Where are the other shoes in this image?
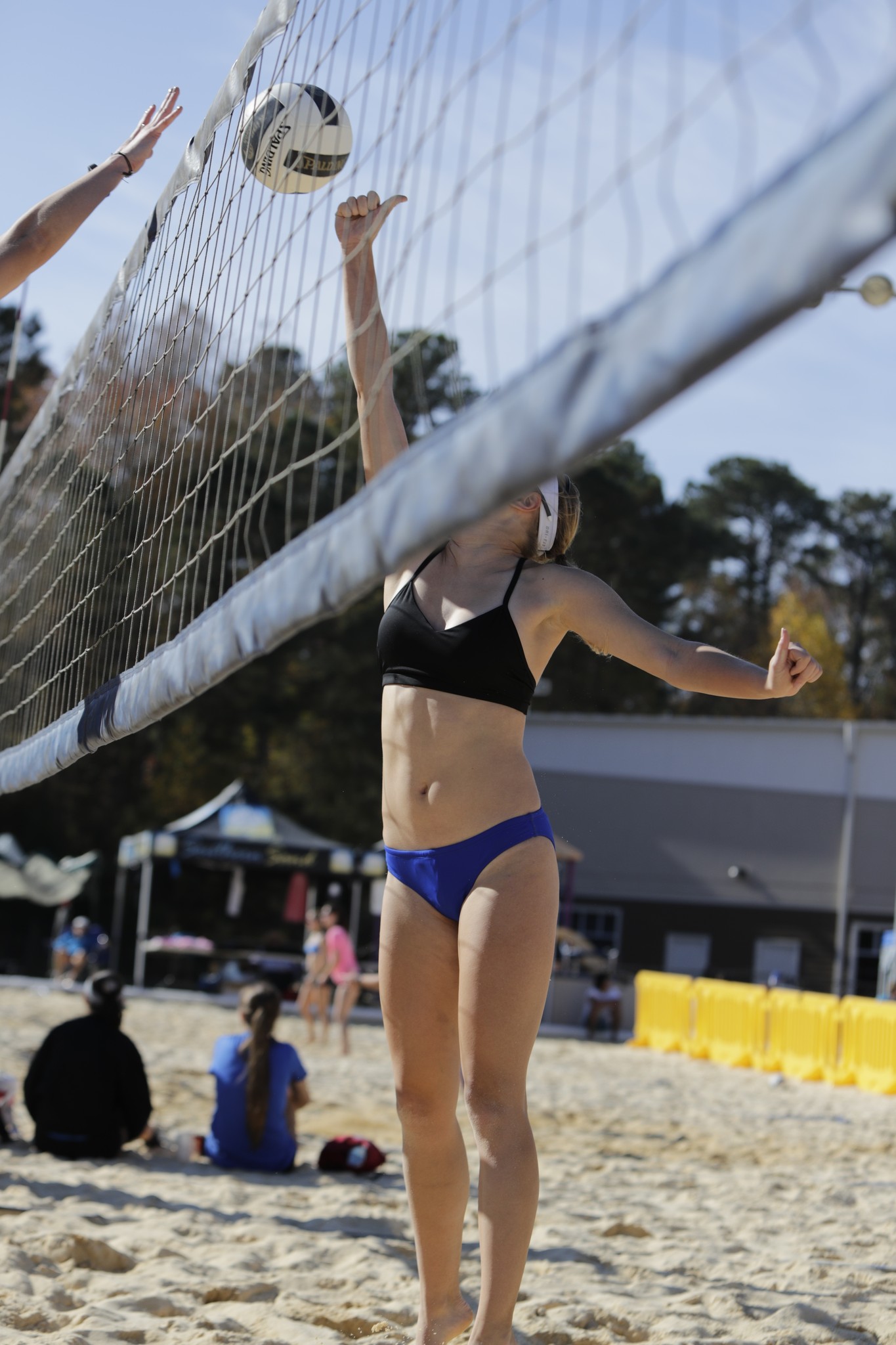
[195,1136,206,1155]
[145,1126,161,1148]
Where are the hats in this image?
[84,969,124,1010]
[535,475,559,553]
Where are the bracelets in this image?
[87,164,112,197]
[114,152,134,183]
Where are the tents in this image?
[0,776,382,994]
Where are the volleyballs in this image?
[240,80,354,196]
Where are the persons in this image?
[0,901,621,1174]
[335,191,823,1345]
[0,86,183,299]
